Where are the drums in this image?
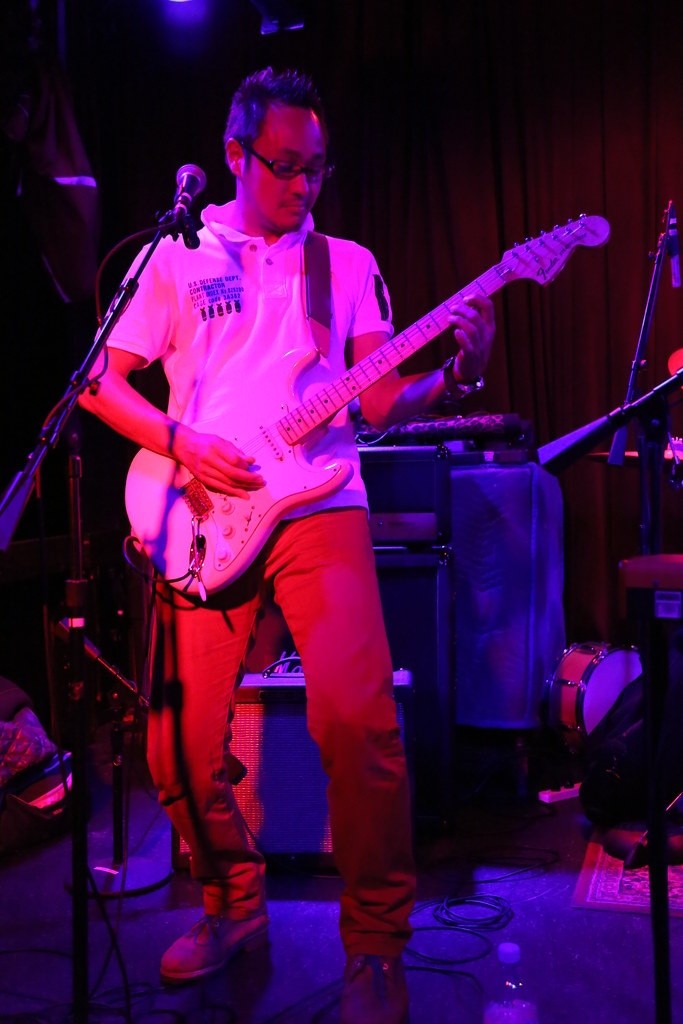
[547,639,649,755]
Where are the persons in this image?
[77,66,495,1024]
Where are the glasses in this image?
[240,141,331,180]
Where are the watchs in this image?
[442,355,486,404]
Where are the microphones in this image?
[173,163,207,220]
[666,205,681,288]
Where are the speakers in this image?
[172,462,566,875]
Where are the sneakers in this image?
[341,954,408,1024]
[160,905,269,985]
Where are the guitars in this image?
[123,209,613,601]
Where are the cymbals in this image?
[586,448,683,470]
